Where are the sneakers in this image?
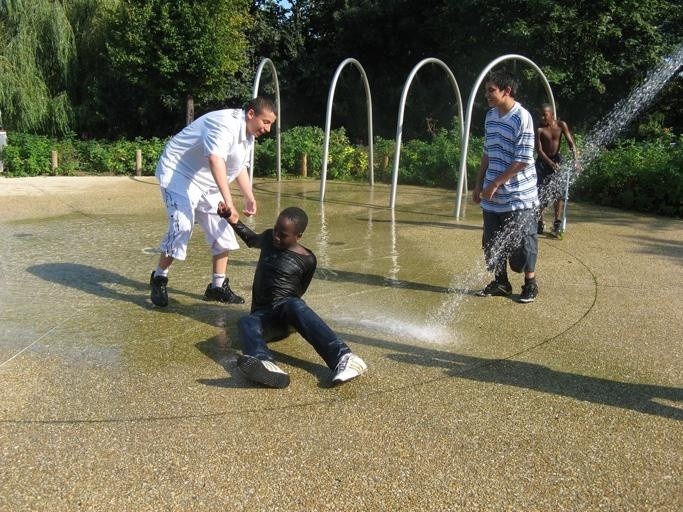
[202,277,245,304]
[537,221,544,234]
[331,353,368,387]
[517,279,539,304]
[553,220,561,233]
[236,353,291,390]
[474,280,512,297]
[149,269,169,308]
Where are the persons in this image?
[149,97,278,305]
[535,103,581,240]
[217,199,370,389]
[472,69,541,303]
